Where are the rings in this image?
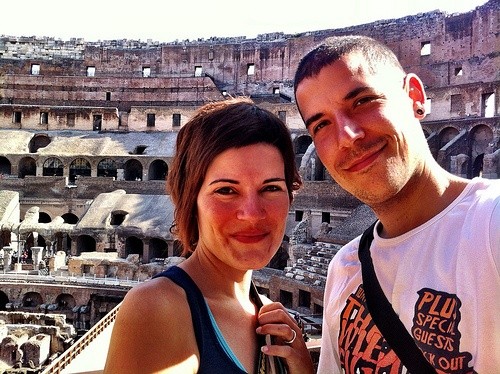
[284,327,297,345]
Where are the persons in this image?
[98,99,317,373]
[293,32,500,374]
